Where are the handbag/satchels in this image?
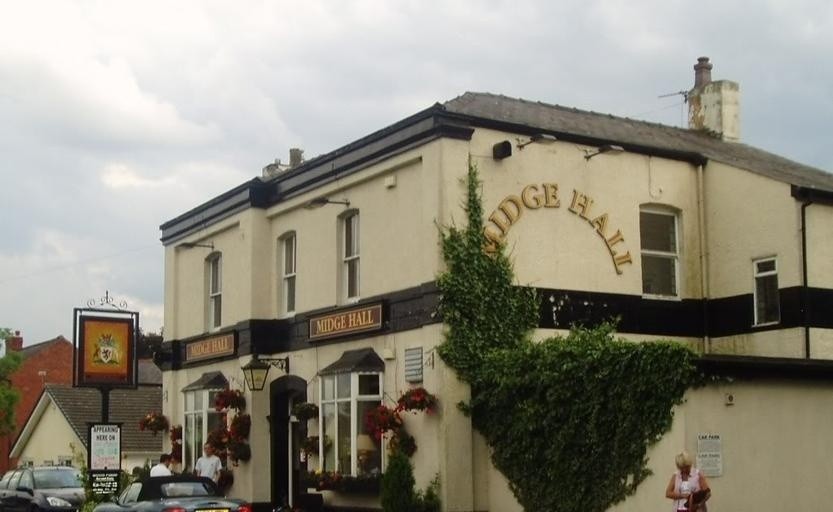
[683,488,709,512]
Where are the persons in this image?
[149,453,182,478]
[666,451,711,512]
[195,442,223,485]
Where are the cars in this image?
[0,467,88,512]
[93,474,252,512]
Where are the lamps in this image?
[241,354,290,391]
[302,198,351,208]
[174,242,214,251]
[584,144,624,162]
[517,134,557,150]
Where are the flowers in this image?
[139,390,437,456]
